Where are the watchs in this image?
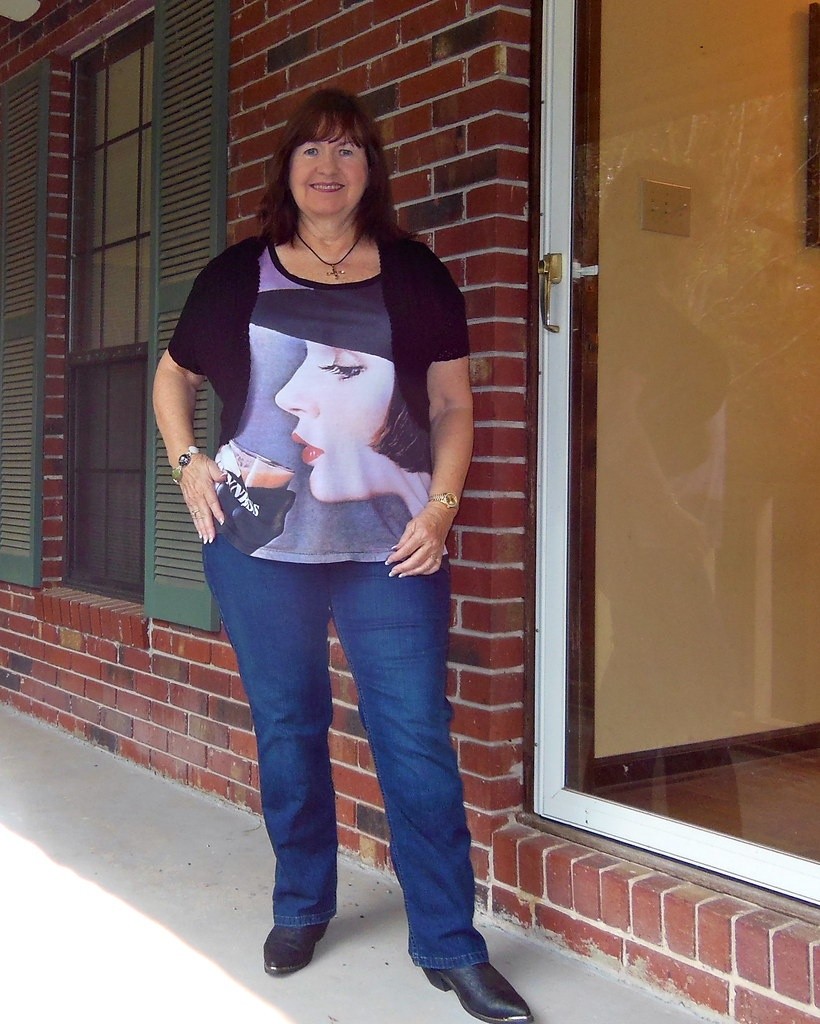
[428,492,459,511]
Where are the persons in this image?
[152,87,532,1022]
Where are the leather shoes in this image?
[421,961,535,1023]
[263,919,331,975]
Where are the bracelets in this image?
[171,444,201,484]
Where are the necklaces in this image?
[296,229,365,281]
